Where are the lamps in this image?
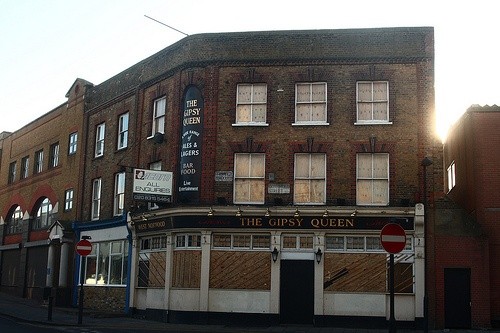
[351,209,359,217]
[265,207,272,216]
[323,209,329,217]
[294,207,300,217]
[142,212,151,221]
[236,207,243,216]
[314,247,323,264]
[271,247,278,263]
[208,207,215,216]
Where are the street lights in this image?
[418,154,432,333]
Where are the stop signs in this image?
[75,240,92,256]
[381,223,406,253]
